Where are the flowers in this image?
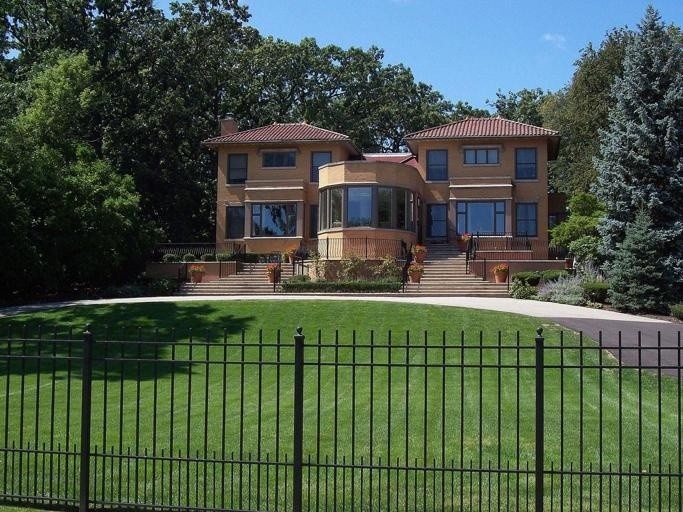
[189,265,205,272]
[411,245,427,253]
[494,263,509,271]
[265,262,282,271]
[409,261,425,271]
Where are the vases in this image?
[409,271,422,283]
[266,271,281,282]
[414,253,426,262]
[495,271,507,282]
[192,272,203,282]
